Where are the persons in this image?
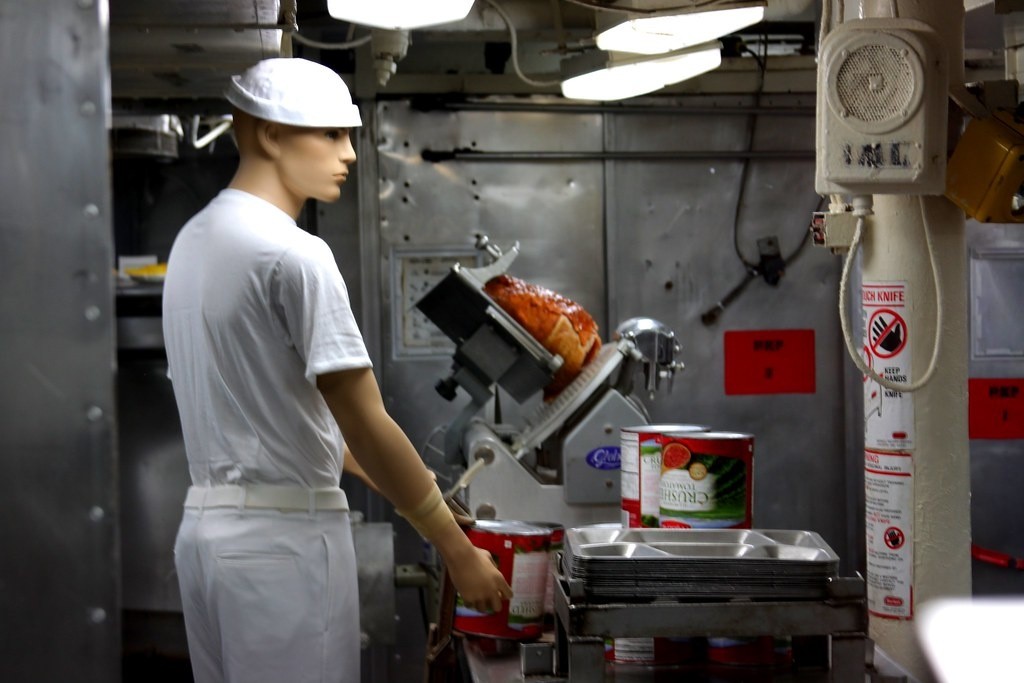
[161,55,515,683]
